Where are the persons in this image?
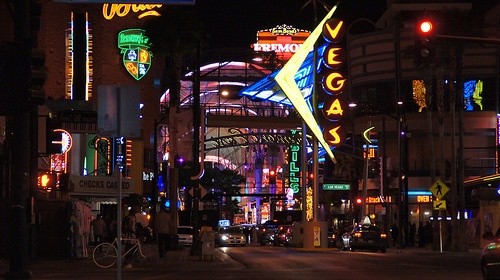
[90,205,172,260]
[383,220,433,249]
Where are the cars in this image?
[176,225,193,246]
[336,216,388,252]
[241,208,338,247]
[217,220,246,246]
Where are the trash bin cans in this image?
[201,230,215,262]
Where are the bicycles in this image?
[92,235,159,269]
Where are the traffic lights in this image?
[417,18,436,60]
